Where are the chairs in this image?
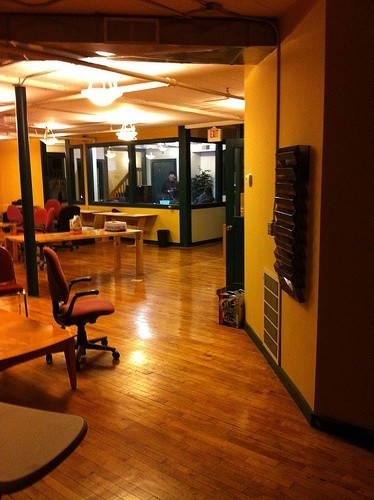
[43,246,120,372]
[3,199,81,251]
[0,246,28,318]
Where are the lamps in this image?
[81,80,123,107]
[115,123,138,141]
[39,129,58,146]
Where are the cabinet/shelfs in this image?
[126,185,153,203]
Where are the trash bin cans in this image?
[217,287,244,328]
[157,230,170,247]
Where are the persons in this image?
[193,187,214,203]
[161,172,178,200]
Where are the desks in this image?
[0,308,79,390]
[0,403,88,495]
[79,209,157,247]
[5,228,144,277]
[0,222,18,262]
